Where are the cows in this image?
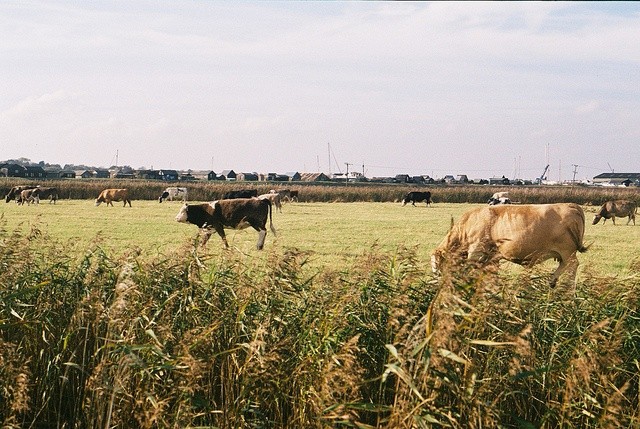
[257,193,282,213]
[94,188,132,207]
[290,190,298,203]
[432,202,594,285]
[175,199,277,250]
[36,187,58,204]
[16,188,40,206]
[268,189,290,205]
[159,187,188,203]
[591,199,638,226]
[401,191,433,208]
[6,186,39,203]
[222,188,258,198]
